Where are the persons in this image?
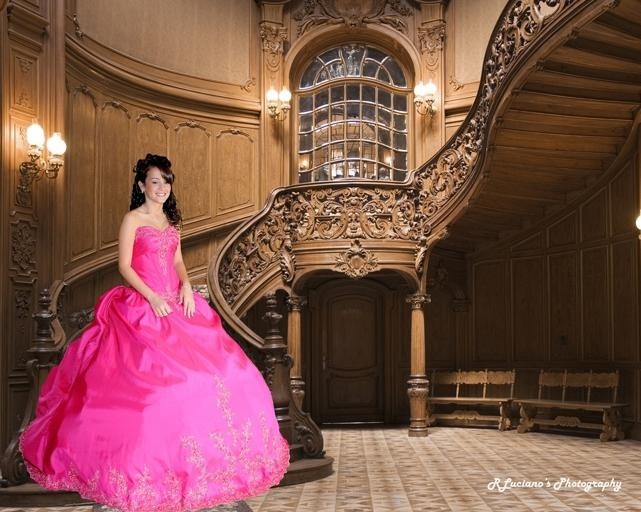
[18,154,291,511]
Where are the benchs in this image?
[428,367,517,431]
[512,370,633,441]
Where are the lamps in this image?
[266,87,291,121]
[17,123,67,193]
[413,78,437,117]
